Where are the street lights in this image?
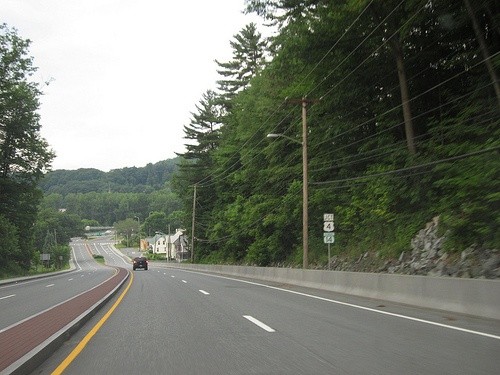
[155,231,170,261]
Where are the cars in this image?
[131,257,147,270]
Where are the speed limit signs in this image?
[323,221,334,244]
